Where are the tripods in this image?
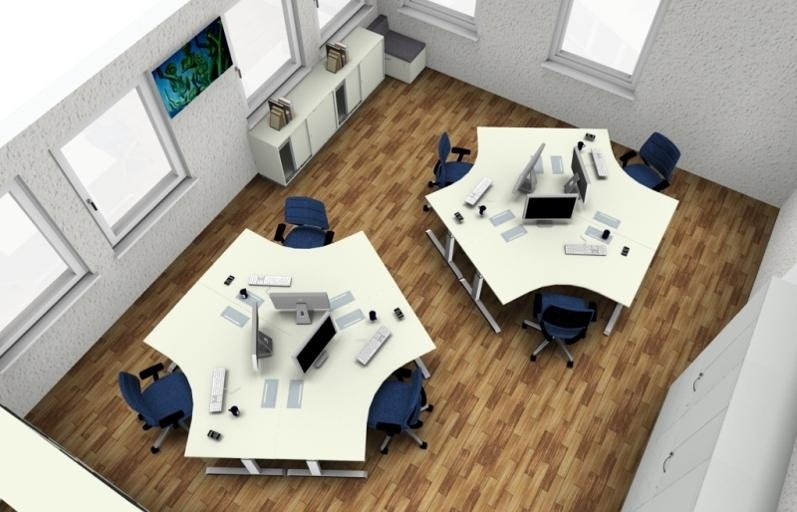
[512,142,546,195]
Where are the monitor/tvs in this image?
[251,302,273,371]
[269,292,332,325]
[521,193,579,228]
[563,146,591,204]
[291,311,337,376]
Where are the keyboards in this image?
[209,367,226,412]
[465,177,492,205]
[248,273,292,287]
[591,147,609,177]
[566,243,608,256]
[355,324,391,365]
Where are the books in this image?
[268,97,294,130]
[326,42,348,74]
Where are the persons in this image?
[156,31,219,95]
